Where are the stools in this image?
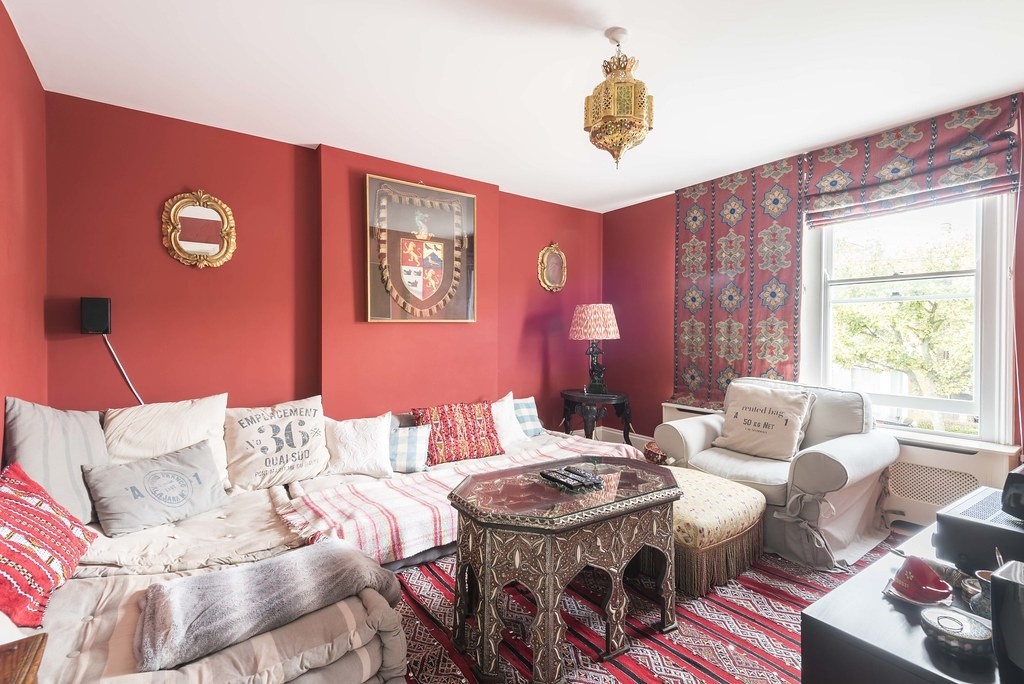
[634,465,767,597]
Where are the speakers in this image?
[79,296,112,334]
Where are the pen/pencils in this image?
[996,547,1004,567]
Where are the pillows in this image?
[712,381,816,462]
[0,461,100,630]
[5,396,111,525]
[514,396,546,436]
[388,423,432,473]
[81,439,230,537]
[224,394,331,496]
[322,410,394,478]
[491,391,531,448]
[411,399,505,467]
[99,392,232,489]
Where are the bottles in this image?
[584,385,587,394]
[969,570,993,620]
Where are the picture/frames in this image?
[364,173,477,324]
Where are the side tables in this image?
[561,388,633,447]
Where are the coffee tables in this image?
[446,456,685,684]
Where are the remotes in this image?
[541,466,605,490]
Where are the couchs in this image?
[654,376,901,574]
[0,413,652,684]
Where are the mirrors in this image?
[161,189,237,269]
[537,242,567,292]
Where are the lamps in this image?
[585,27,654,171]
[569,303,620,395]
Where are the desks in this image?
[800,519,1024,684]
